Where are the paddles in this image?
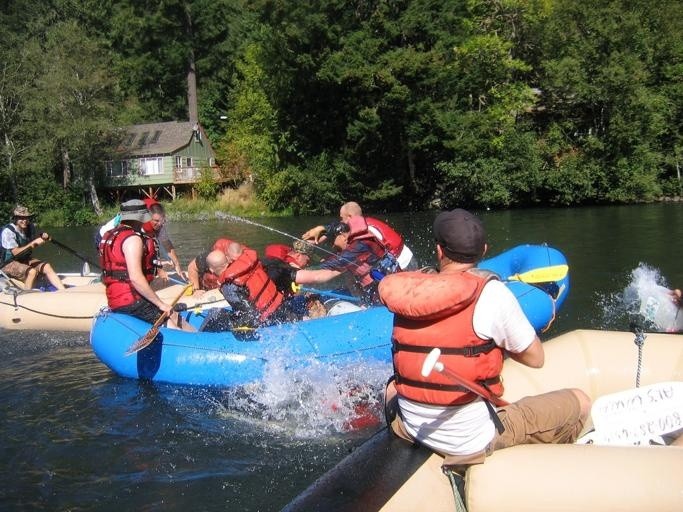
[232,326,256,333]
[125,283,195,356]
[502,264,569,283]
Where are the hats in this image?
[293,240,316,255]
[321,223,349,237]
[120,199,153,223]
[13,206,35,217]
[432,209,485,263]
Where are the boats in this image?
[270,324,683,512]
[0,266,223,335]
[86,240,572,394]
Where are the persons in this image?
[94,199,419,331]
[384,208,591,472]
[0,207,65,291]
